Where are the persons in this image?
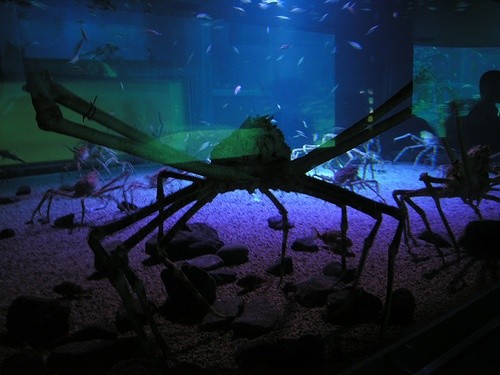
[462,70,500,161]
